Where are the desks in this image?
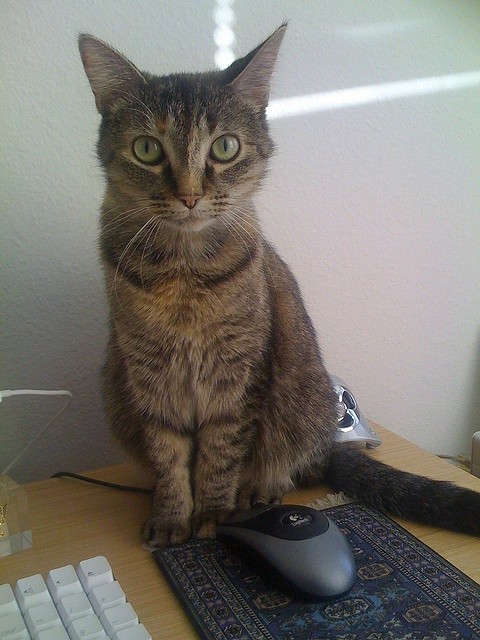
[0,418,479,639]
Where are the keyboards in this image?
[0,555,152,639]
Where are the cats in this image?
[77,21,480,548]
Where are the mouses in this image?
[216,503,359,602]
